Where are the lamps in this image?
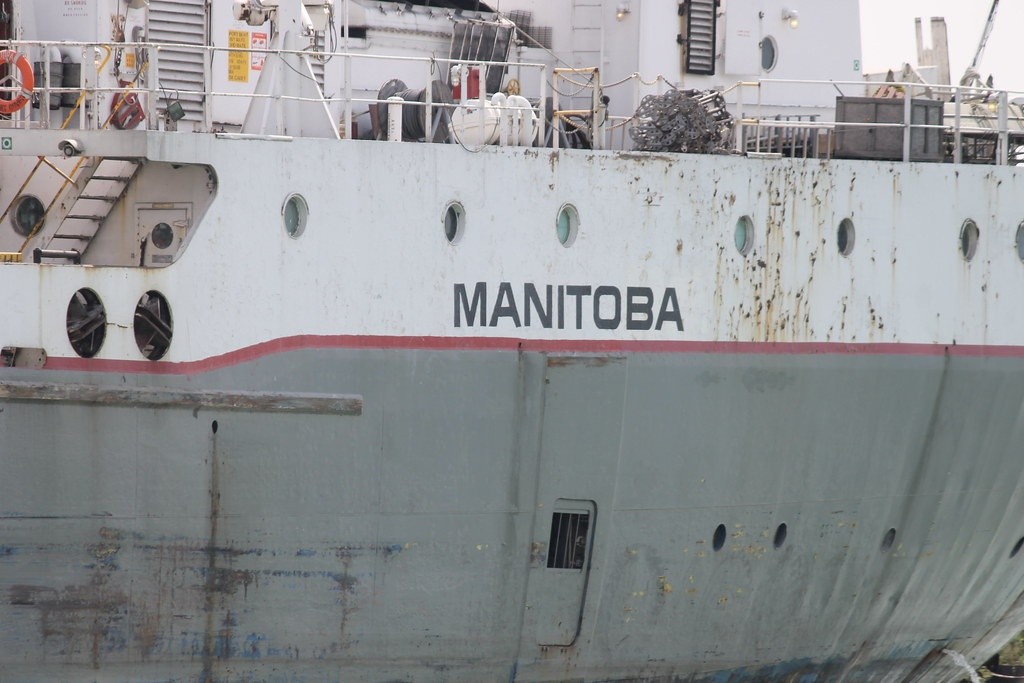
[783,8,799,28]
[165,100,184,122]
[616,2,630,17]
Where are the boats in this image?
[0,0,1024,683]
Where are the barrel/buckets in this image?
[166,91,184,121]
[62,55,81,108]
[32,61,63,110]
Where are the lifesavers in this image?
[0,49,35,114]
